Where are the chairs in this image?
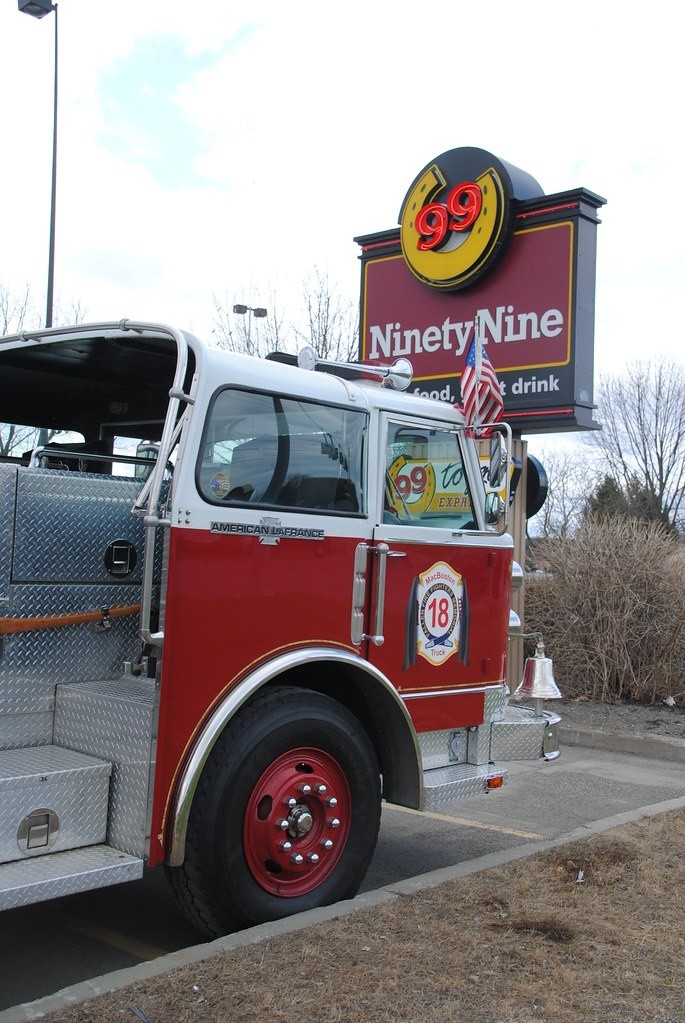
[19,441,109,474]
[233,438,345,508]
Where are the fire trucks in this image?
[0,321,563,947]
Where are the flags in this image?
[459,327,504,436]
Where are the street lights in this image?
[16,0,61,448]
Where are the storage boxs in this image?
[0,745,114,865]
